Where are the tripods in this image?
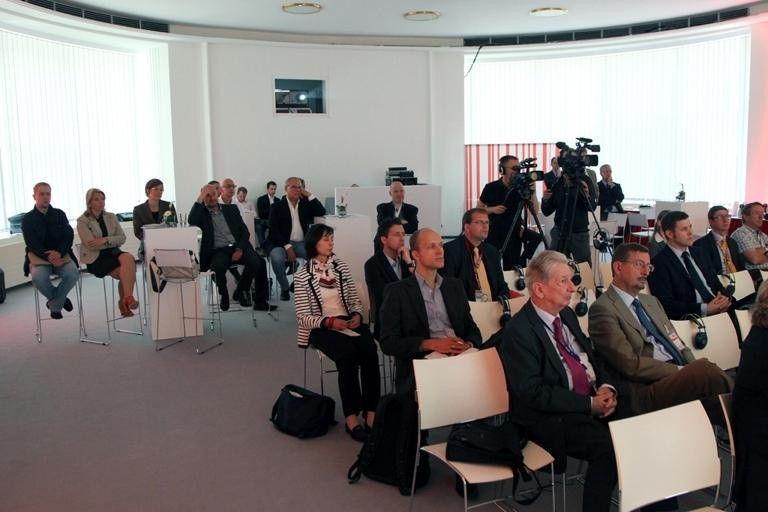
[498,201,550,267]
[556,183,614,257]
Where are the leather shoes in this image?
[279,281,294,299]
[232,289,252,307]
[455,473,478,501]
[46,301,62,319]
[118,296,139,315]
[345,421,373,442]
[220,293,229,311]
[63,297,73,311]
[253,301,277,311]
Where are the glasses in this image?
[712,215,732,219]
[622,260,655,272]
[152,187,164,192]
[474,219,490,225]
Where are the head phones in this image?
[680,313,707,349]
[576,286,588,316]
[496,295,511,328]
[718,270,735,295]
[513,265,525,291]
[567,261,581,285]
[497,156,505,175]
[592,228,610,252]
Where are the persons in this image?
[436,208,511,304]
[494,250,678,512]
[77,189,139,318]
[588,243,737,430]
[379,229,482,500]
[327,316,336,330]
[365,218,416,322]
[375,182,420,234]
[732,278,768,512]
[133,179,178,263]
[187,178,326,311]
[541,156,624,268]
[646,202,768,320]
[21,183,80,319]
[476,156,538,270]
[293,224,381,443]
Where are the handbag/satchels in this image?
[155,250,199,278]
[273,383,335,438]
[445,422,529,465]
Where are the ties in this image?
[682,251,715,302]
[632,299,684,365]
[720,239,737,272]
[474,247,493,302]
[553,318,591,395]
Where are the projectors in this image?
[277,90,308,105]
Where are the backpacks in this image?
[357,393,430,487]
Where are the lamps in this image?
[282,2,321,14]
[530,8,568,17]
[405,10,440,21]
